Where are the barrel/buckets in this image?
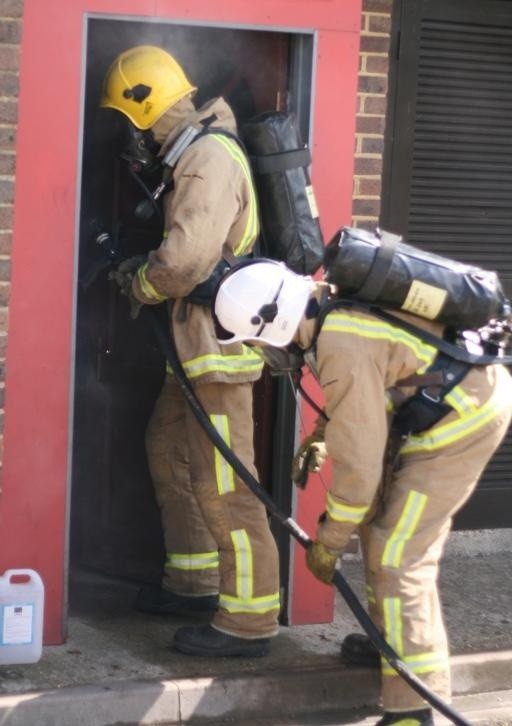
[0,569,45,666]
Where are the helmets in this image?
[99,44,197,130]
[212,256,310,348]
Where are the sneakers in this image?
[173,623,271,657]
[339,630,384,667]
[137,581,219,617]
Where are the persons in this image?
[209,256,512,726]
[95,45,281,661]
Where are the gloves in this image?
[290,424,327,489]
[304,539,342,584]
[107,255,151,321]
